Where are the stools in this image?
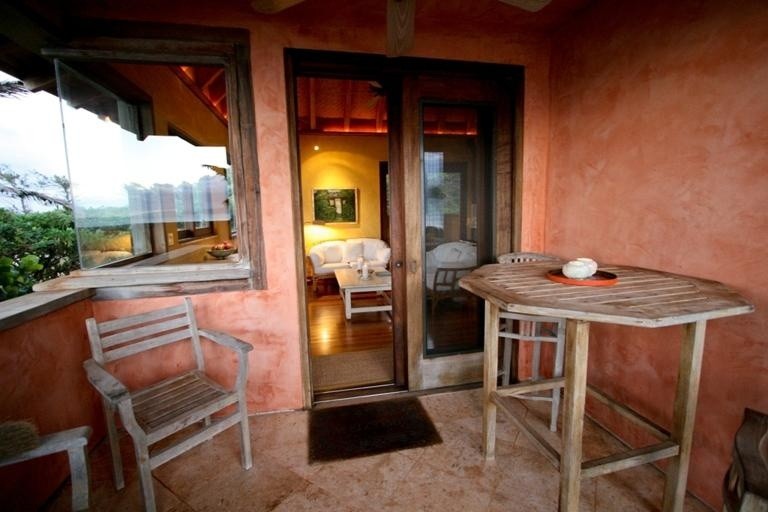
[0,425,93,512]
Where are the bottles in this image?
[356,256,369,280]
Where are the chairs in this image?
[425,265,477,322]
[496,252,566,433]
[81,296,253,512]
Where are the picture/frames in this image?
[312,188,359,224]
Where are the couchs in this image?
[305,238,392,292]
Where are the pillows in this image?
[323,239,383,262]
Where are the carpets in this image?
[307,394,443,466]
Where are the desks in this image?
[458,257,755,512]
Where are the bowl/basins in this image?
[206,247,237,260]
[574,257,598,278]
[559,260,588,280]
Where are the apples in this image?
[211,240,233,250]
